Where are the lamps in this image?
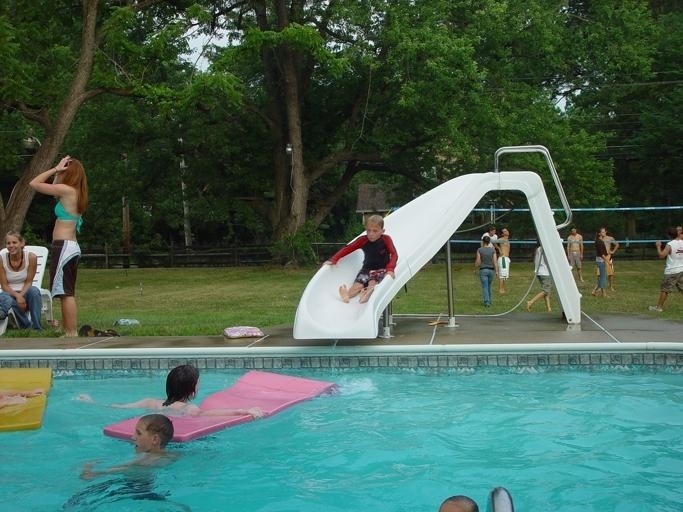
[285,143,293,153]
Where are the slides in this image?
[292,172,494,339]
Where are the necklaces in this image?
[8,254,24,271]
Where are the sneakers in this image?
[647,305,662,313]
[526,300,532,312]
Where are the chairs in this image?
[0,245,53,336]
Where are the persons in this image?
[476,224,619,312]
[437,496,480,511]
[324,215,398,302]
[30,156,87,337]
[648,226,683,312]
[81,414,193,480]
[0,230,41,332]
[77,364,265,420]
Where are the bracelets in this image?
[54,167,59,173]
